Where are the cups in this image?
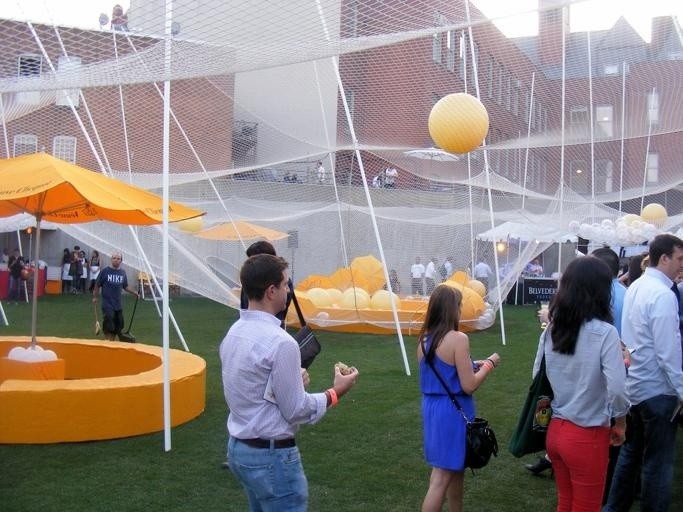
[540,300,550,308]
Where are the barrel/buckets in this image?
[0,271,17,300]
[24,265,46,298]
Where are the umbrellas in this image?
[0,147,207,349]
[195,220,291,288]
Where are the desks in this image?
[522,275,559,305]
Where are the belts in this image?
[240,436,297,449]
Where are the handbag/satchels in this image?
[465,416,498,470]
[293,324,321,369]
[509,370,553,459]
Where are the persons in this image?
[222,241,294,472]
[385,163,398,189]
[377,167,386,188]
[316,161,325,186]
[91,252,140,340]
[373,176,379,188]
[390,256,495,300]
[1,248,9,263]
[60,245,101,295]
[7,249,25,306]
[526,234,681,512]
[218,253,359,511]
[417,284,500,510]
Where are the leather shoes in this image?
[524,456,552,474]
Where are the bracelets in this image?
[327,387,340,410]
[484,359,495,372]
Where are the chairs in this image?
[161,273,180,296]
[137,272,157,297]
